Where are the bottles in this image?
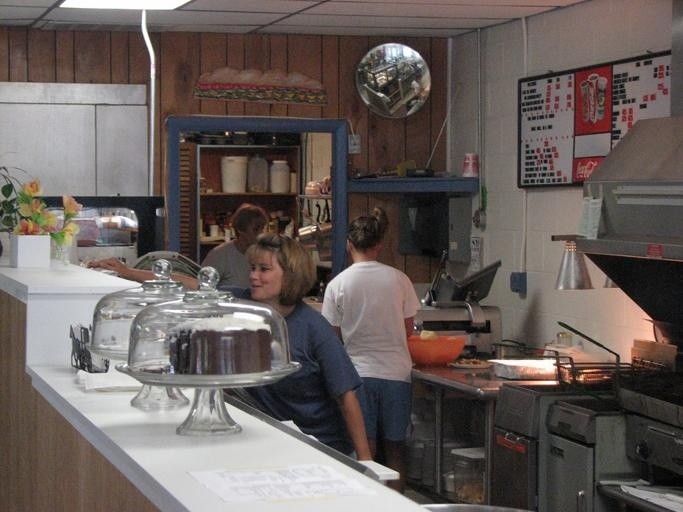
[247,155,268,192]
[270,160,290,194]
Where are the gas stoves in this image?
[618,358,683,407]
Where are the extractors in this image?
[552,117,682,323]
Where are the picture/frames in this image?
[518,49,672,190]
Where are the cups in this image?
[580,73,608,123]
[462,153,479,178]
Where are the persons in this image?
[320,206,421,493]
[88,234,371,460]
[411,73,425,96]
[199,203,268,287]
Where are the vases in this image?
[9,233,51,268]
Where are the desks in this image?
[404,352,613,506]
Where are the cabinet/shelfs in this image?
[194,143,301,267]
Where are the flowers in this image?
[12,178,83,249]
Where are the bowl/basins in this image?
[407,335,468,366]
